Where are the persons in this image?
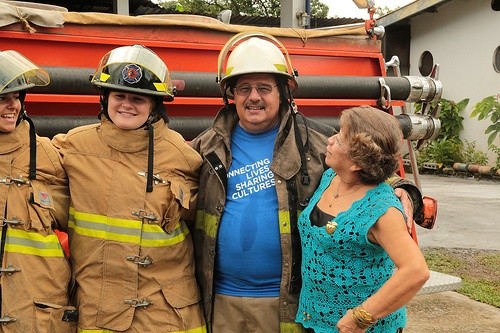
[191,32,424,332]
[0,49,72,332]
[293,105,431,333]
[51,44,207,333]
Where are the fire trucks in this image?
[0,0,444,258]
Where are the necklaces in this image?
[328,180,365,207]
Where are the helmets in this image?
[0,51,36,94]
[90,44,176,103]
[216,29,299,101]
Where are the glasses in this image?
[232,80,279,95]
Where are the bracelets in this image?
[351,304,379,330]
